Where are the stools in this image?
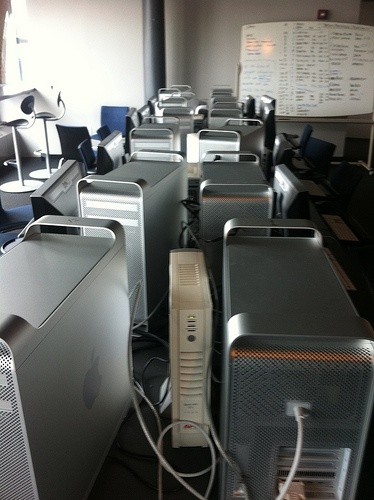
[0,95,44,194]
[28,89,67,181]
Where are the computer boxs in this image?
[3,83,373,499]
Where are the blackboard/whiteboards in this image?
[234,20,374,125]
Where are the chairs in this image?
[298,159,363,219]
[90,105,130,145]
[292,136,337,185]
[0,195,34,235]
[78,137,101,175]
[55,123,94,176]
[286,124,314,160]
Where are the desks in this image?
[0,84,36,102]
